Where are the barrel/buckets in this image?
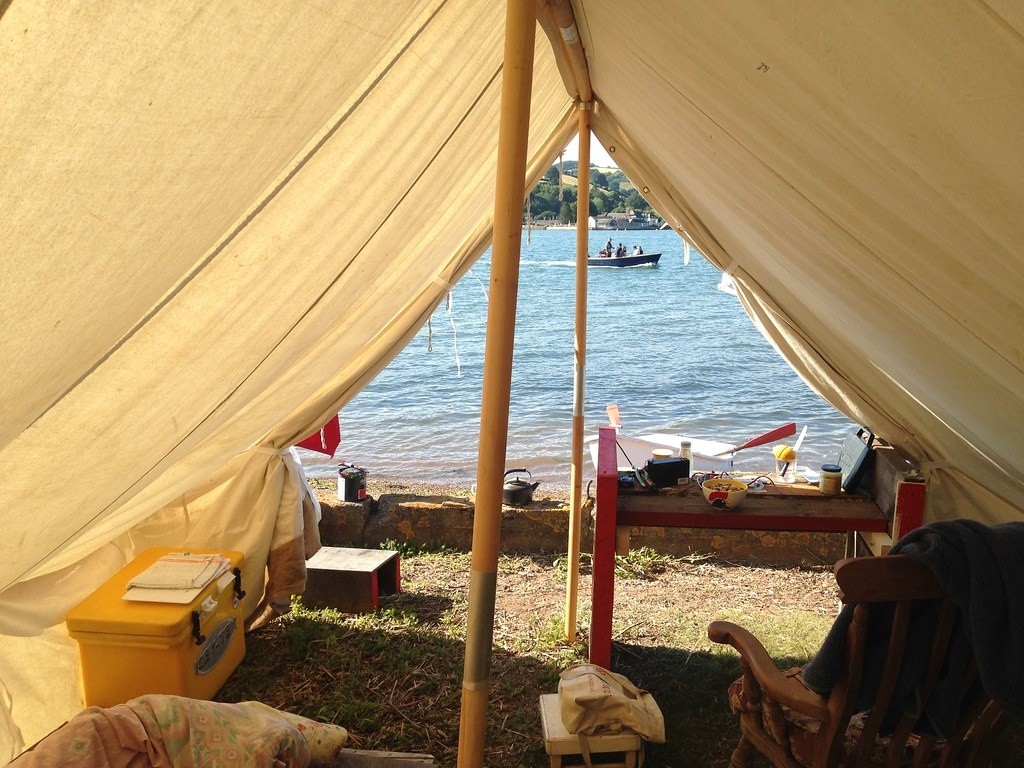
[337,463,369,503]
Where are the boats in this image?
[581,238,660,268]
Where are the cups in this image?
[606,404,620,425]
[651,448,674,460]
[775,455,799,484]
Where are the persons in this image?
[615,243,643,257]
[605,237,615,257]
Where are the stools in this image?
[303,547,402,614]
[539,695,641,768]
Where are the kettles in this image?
[502,467,540,505]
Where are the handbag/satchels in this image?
[557,663,665,768]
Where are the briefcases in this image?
[837,425,875,494]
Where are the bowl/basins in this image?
[702,478,748,510]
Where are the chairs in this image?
[707,553,1024,768]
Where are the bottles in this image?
[677,441,693,480]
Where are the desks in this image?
[590,428,923,672]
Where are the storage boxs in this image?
[67,548,250,711]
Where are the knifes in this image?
[778,425,808,477]
